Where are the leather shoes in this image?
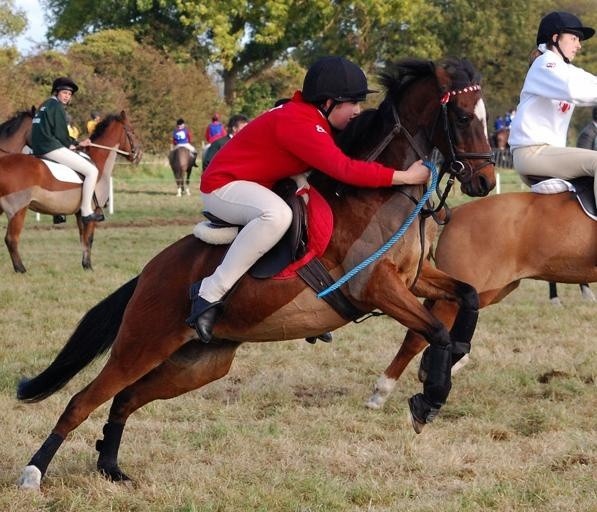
[80,212,104,223]
[190,279,219,344]
[317,332,332,343]
[53,215,66,225]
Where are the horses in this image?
[1,106,142,273]
[166,144,198,197]
[362,178,596,409]
[14,58,494,495]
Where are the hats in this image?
[51,77,79,95]
[302,56,380,103]
[536,10,595,44]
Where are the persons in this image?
[188,55,432,344]
[507,11,597,208]
[87,109,101,138]
[504,108,515,129]
[65,112,79,140]
[172,118,199,168]
[576,105,597,151]
[202,113,227,164]
[31,76,105,224]
[202,115,250,172]
[490,115,504,149]
